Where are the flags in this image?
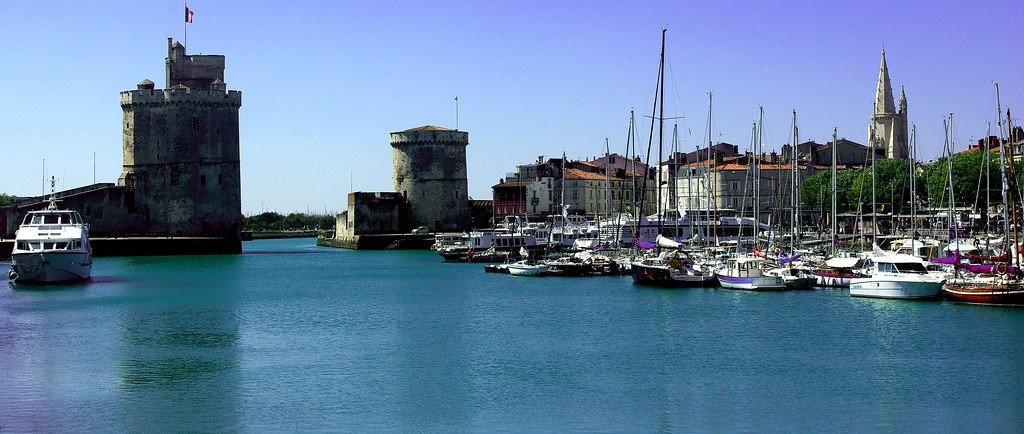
[185,7,194,24]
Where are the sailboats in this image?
[431,23,1024,308]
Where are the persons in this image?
[467,248,473,258]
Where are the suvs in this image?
[412,225,429,235]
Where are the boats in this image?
[7,158,94,283]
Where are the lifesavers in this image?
[995,262,1008,275]
